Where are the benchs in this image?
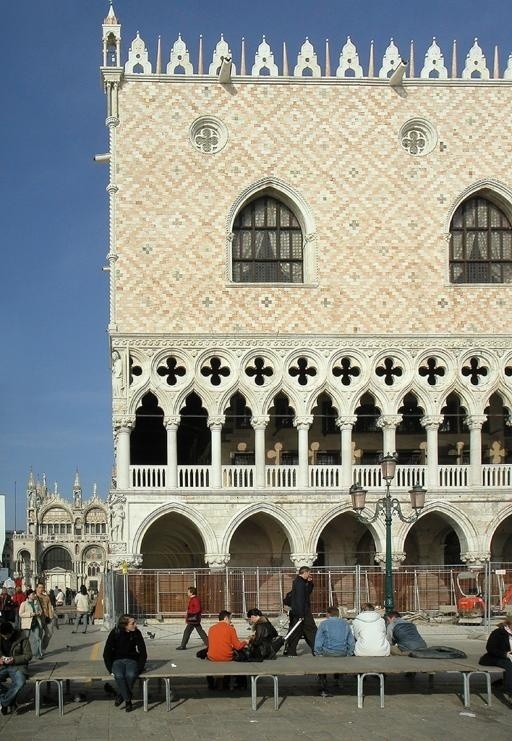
[1,651,511,714]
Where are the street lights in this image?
[349,451,429,637]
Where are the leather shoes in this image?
[176,646,185,650]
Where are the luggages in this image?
[271,618,303,651]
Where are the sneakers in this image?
[126,701,132,712]
[207,682,247,691]
[115,694,123,706]
[321,685,342,693]
[1,705,7,715]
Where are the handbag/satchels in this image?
[283,591,291,606]
[186,614,198,622]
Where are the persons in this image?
[501,585,512,622]
[314,607,355,689]
[1,622,34,716]
[282,579,298,657]
[234,609,279,692]
[485,616,512,699]
[1,577,99,662]
[351,603,390,682]
[175,587,209,652]
[385,611,428,681]
[104,613,147,713]
[207,611,251,693]
[287,567,318,657]
